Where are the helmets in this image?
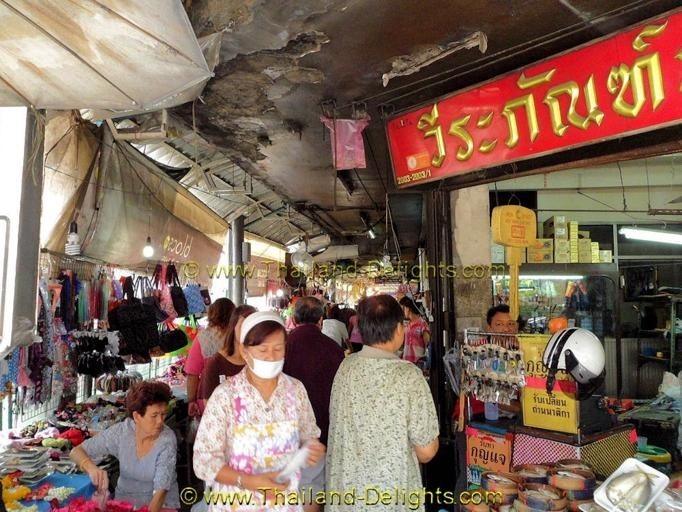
[541,326,607,402]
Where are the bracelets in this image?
[78,457,90,475]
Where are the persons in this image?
[451,303,520,512]
[69,380,181,512]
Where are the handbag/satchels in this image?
[109,263,213,364]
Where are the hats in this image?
[239,310,285,344]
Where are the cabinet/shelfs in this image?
[629,285,681,400]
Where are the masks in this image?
[244,350,285,380]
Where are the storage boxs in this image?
[22,470,95,511]
[509,418,643,481]
[465,408,518,489]
[485,210,613,268]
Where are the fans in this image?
[289,233,317,273]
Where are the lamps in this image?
[618,214,682,246]
[66,176,83,258]
[142,189,155,260]
